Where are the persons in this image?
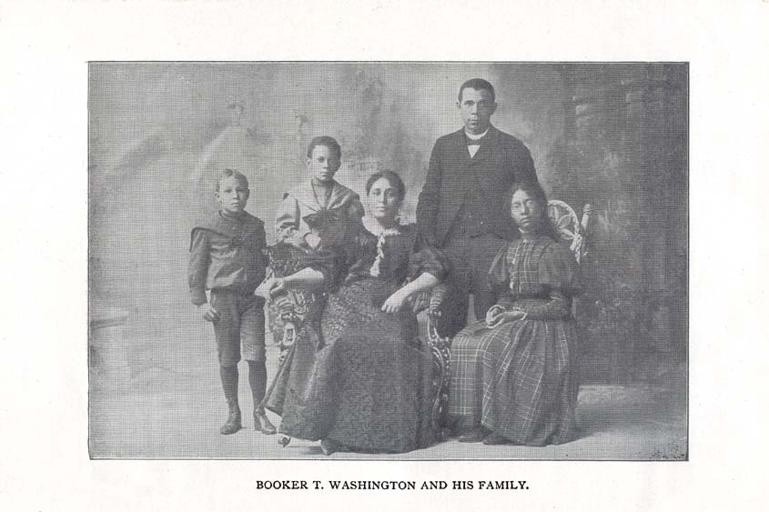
[417,79,547,337]
[261,170,452,456]
[274,136,365,254]
[446,180,586,447]
[187,169,277,435]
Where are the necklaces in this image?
[313,181,332,200]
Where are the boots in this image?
[253,399,276,434]
[220,397,240,434]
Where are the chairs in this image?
[546,197,594,321]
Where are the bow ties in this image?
[466,136,483,145]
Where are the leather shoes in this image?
[458,426,510,444]
